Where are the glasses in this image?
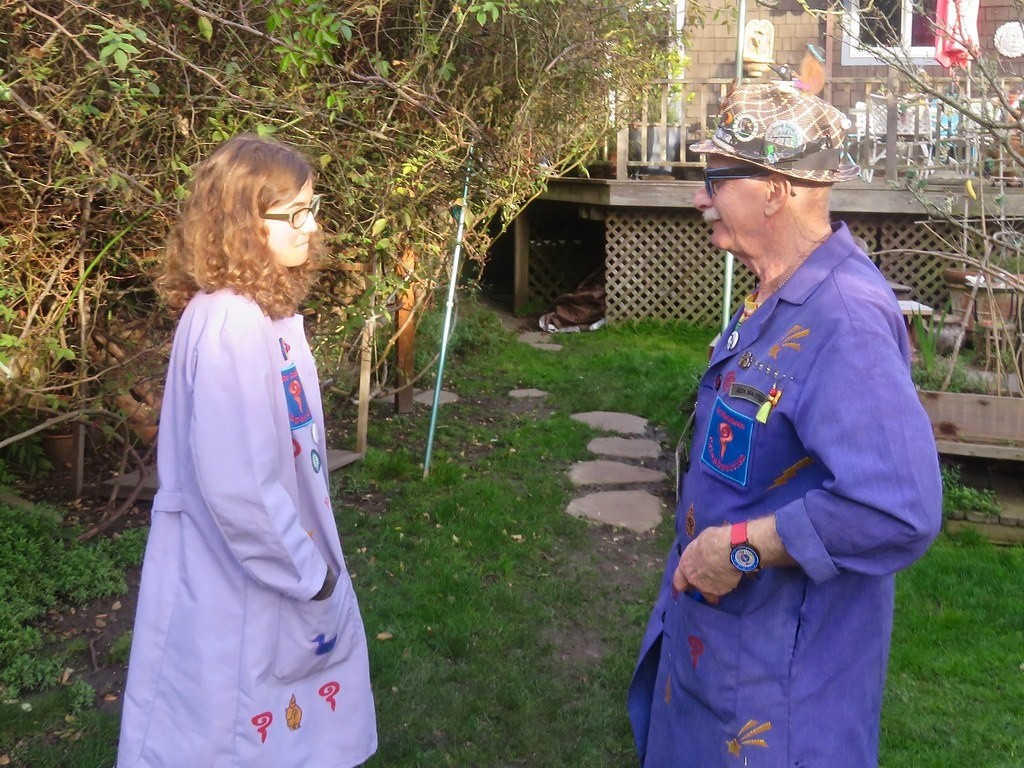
[260,195,322,228]
[702,164,797,198]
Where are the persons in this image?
[626,83,943,768]
[118,137,377,768]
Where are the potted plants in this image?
[625,95,697,175]
[950,485,1000,524]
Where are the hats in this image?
[688,78,861,183]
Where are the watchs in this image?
[729,523,764,574]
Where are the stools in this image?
[970,320,1015,372]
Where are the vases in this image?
[577,137,629,180]
[41,432,74,468]
[923,267,1024,358]
[680,141,706,181]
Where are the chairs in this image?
[855,94,1002,185]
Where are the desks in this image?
[888,282,932,363]
